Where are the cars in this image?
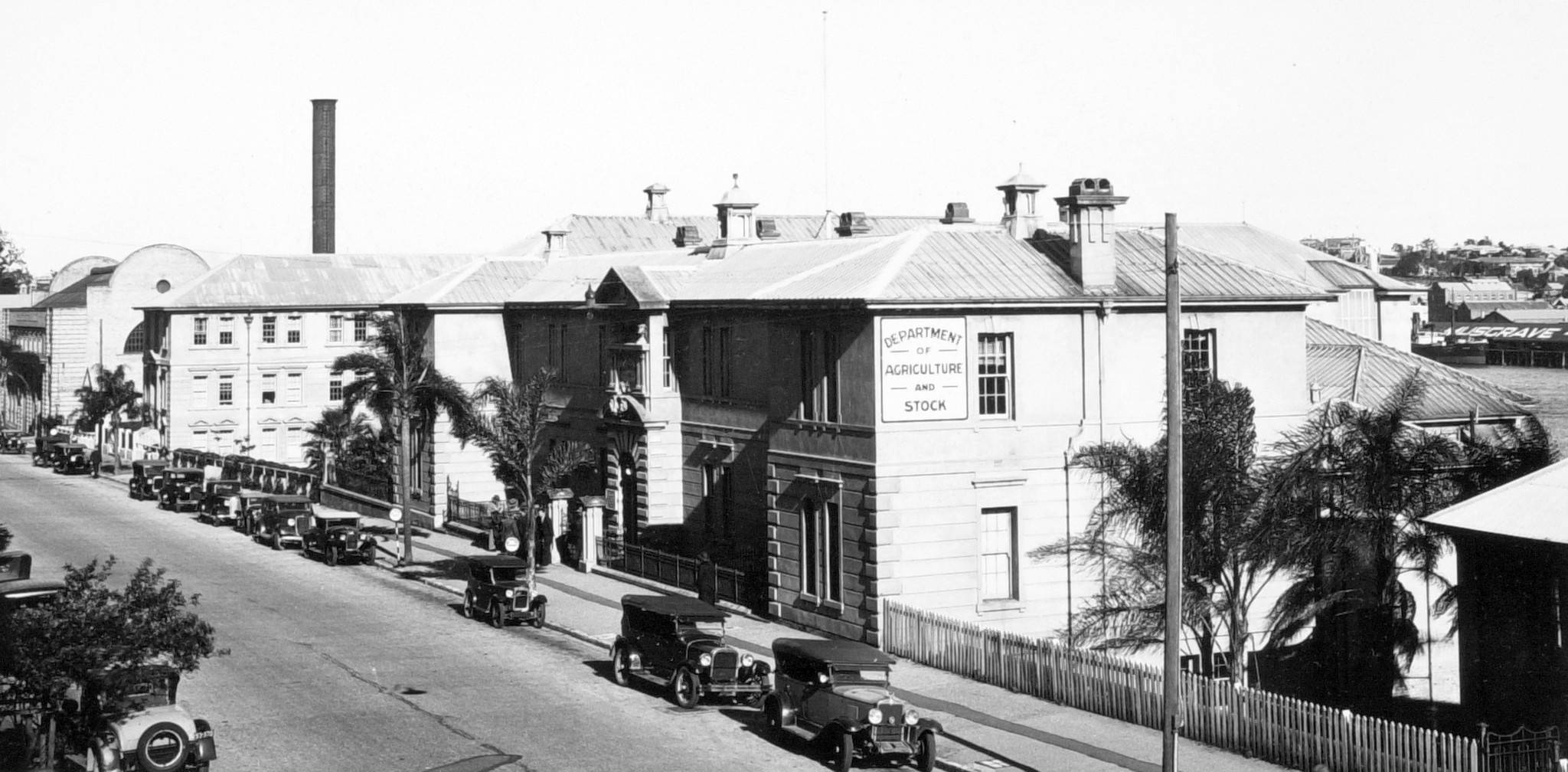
[0,578,73,617]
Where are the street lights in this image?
[243,312,254,456]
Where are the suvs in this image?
[253,495,317,551]
[59,649,219,772]
[129,458,174,500]
[158,467,209,513]
[0,429,26,454]
[462,555,548,629]
[31,436,67,468]
[301,510,378,567]
[196,479,247,527]
[51,443,94,475]
[608,594,773,709]
[764,638,944,772]
[234,492,274,536]
